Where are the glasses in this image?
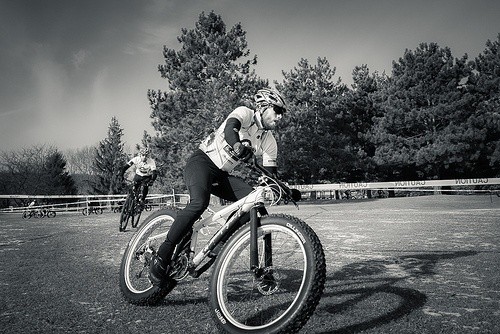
[273,105,285,116]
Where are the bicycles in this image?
[82,203,103,216]
[113,203,123,214]
[115,174,154,232]
[22,208,57,220]
[118,145,327,334]
[144,202,153,212]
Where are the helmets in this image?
[254,87,287,115]
[139,148,149,155]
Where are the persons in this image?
[120,148,157,206]
[148,88,301,290]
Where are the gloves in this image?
[234,142,253,163]
[288,188,302,203]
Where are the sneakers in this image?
[148,252,170,288]
